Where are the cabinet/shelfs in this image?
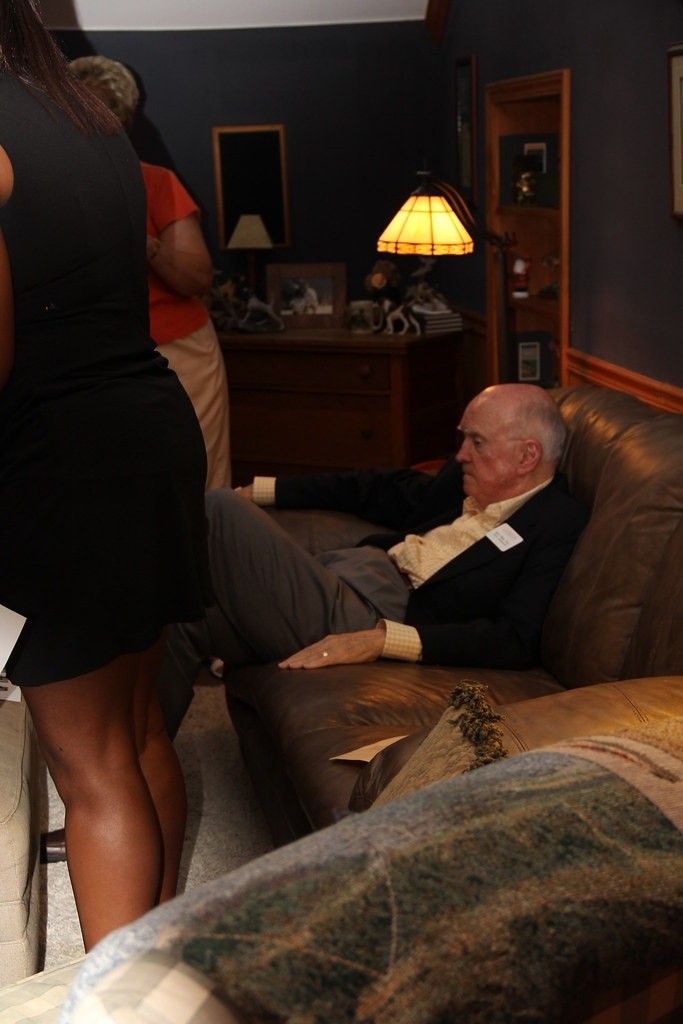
[220,330,454,475]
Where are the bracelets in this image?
[147,239,162,263]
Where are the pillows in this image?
[367,679,509,811]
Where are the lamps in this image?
[370,169,520,373]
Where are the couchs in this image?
[65,387,683,1024]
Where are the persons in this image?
[64,54,232,488]
[1,0,224,959]
[289,277,321,314]
[159,384,593,743]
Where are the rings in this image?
[323,650,330,657]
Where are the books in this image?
[408,307,464,335]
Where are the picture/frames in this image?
[215,126,291,252]
[268,262,349,330]
[667,44,683,216]
